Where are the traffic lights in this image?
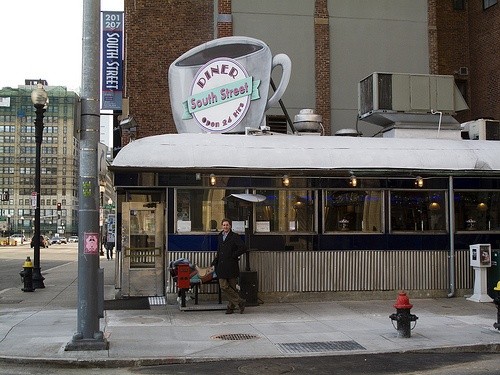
[57,203,61,211]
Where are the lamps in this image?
[415,175,424,188]
[350,175,357,186]
[283,175,290,186]
[210,174,216,186]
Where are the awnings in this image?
[108,133,500,175]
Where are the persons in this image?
[211,219,245,314]
[104,233,115,261]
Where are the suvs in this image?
[30,234,49,248]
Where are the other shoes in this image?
[238,300,247,314]
[225,305,235,314]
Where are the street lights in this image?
[30,77,48,290]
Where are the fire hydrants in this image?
[389,290,418,339]
[19,257,35,292]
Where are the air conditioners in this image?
[458,67,469,76]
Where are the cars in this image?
[6,234,27,243]
[48,235,79,245]
